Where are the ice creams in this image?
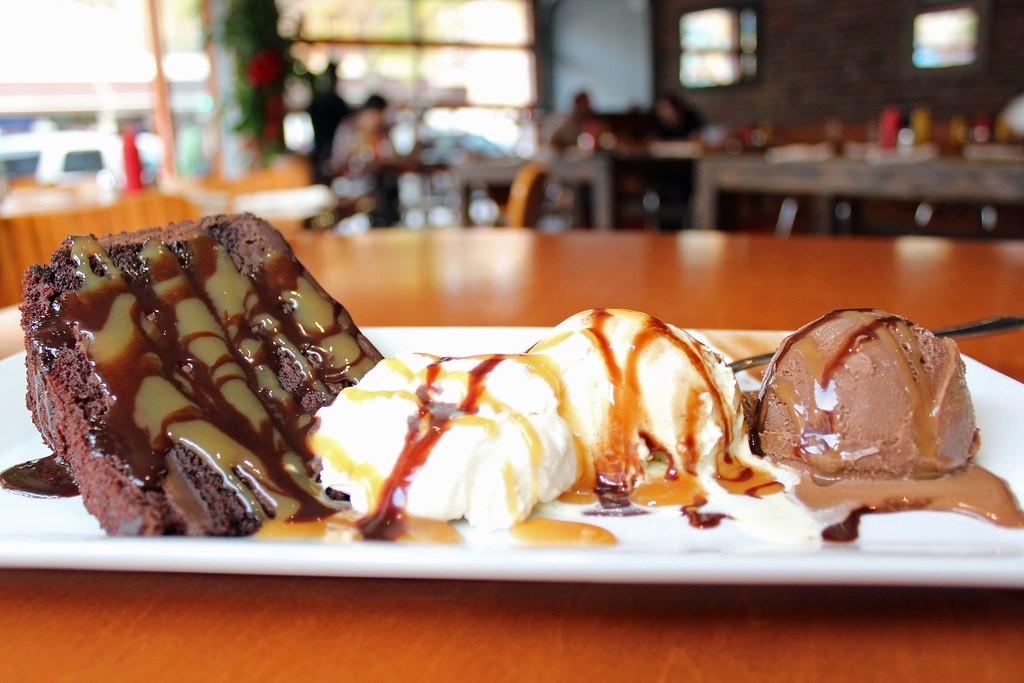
[303,309,981,529]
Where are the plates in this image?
[0,326,1024,586]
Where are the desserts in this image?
[20,208,380,540]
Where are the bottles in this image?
[875,104,1014,149]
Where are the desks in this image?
[694,150,1024,242]
[0,226,1024,683]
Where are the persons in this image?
[330,95,432,231]
[547,87,736,230]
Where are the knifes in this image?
[728,315,1022,377]
